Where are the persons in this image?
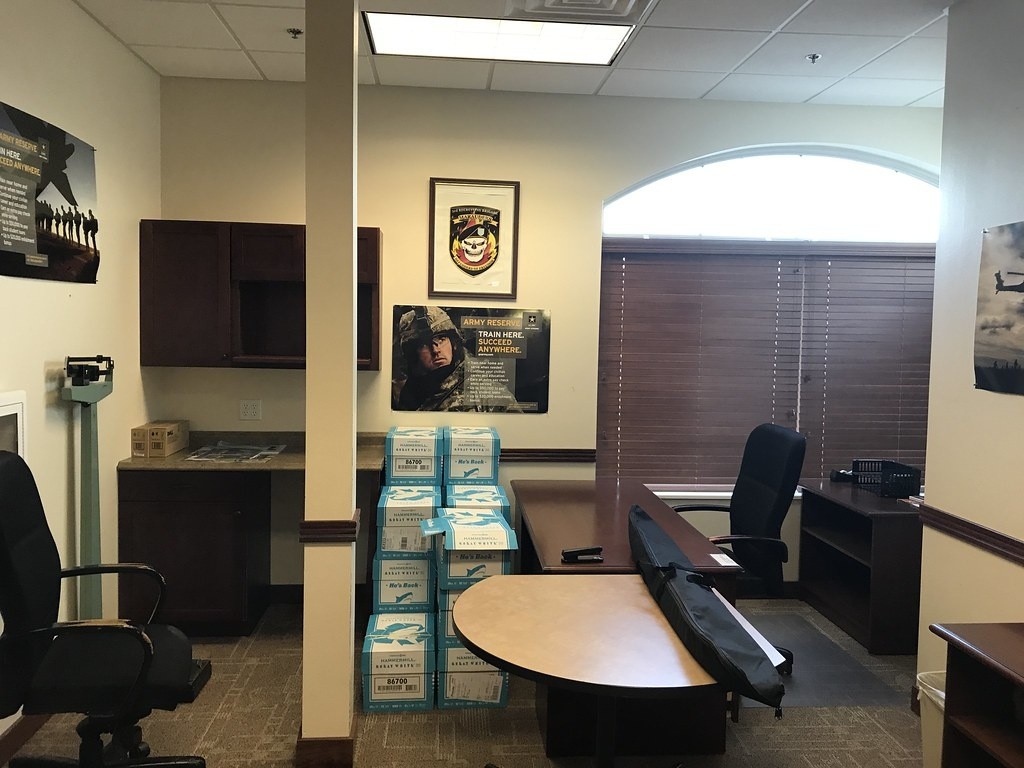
[392,306,523,413]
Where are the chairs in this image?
[0,449,212,768]
[672,422,807,676]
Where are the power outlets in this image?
[239,399,262,421]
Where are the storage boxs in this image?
[361,426,519,712]
[131,419,189,457]
[852,457,921,498]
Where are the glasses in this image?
[418,334,451,353]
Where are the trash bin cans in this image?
[915,671,947,768]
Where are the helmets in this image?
[399,306,461,358]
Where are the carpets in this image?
[736,614,906,709]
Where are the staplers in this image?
[560,545,604,563]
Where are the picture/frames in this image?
[428,177,520,299]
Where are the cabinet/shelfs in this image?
[139,218,381,371]
[798,476,923,656]
[929,622,1024,768]
[118,431,386,637]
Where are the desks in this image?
[451,575,785,768]
[508,476,747,756]
